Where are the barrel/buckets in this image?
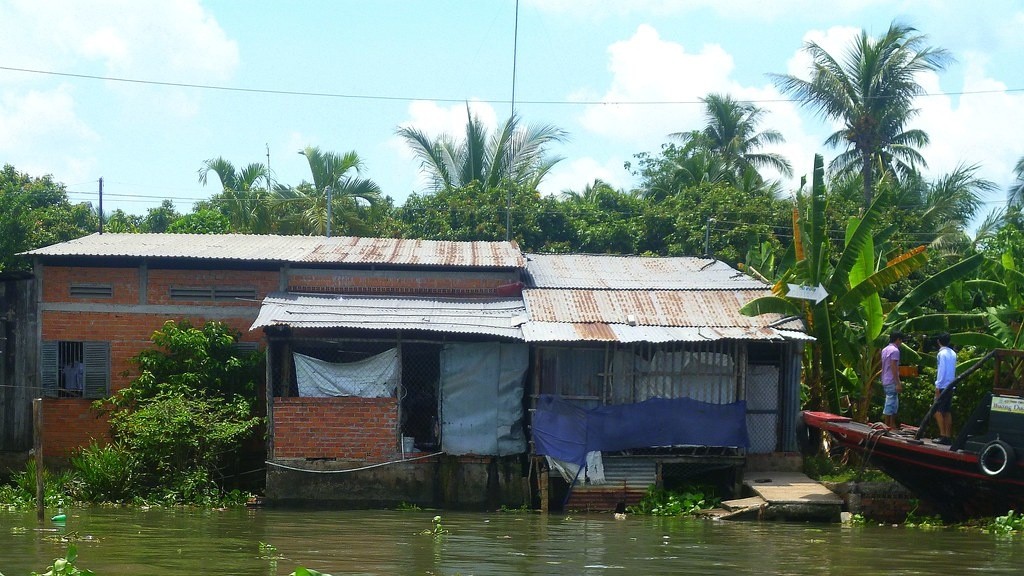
[403,436,414,453]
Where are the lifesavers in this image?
[978,439,1016,480]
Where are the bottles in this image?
[50,514,67,521]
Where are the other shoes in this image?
[943,438,952,444]
[932,436,947,444]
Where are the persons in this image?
[932,332,957,444]
[882,330,903,431]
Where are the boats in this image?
[802,347,1022,528]
